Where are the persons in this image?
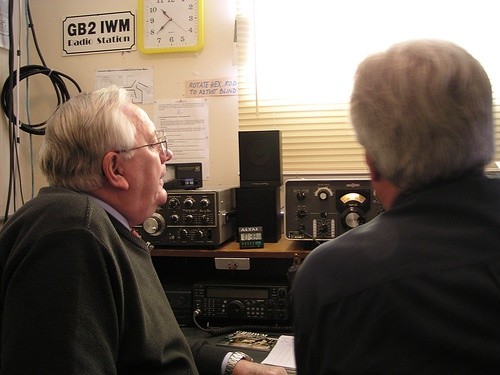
[288,37,500,375]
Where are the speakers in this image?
[238,130,283,187]
[235,187,281,243]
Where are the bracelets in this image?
[0,83,288,375]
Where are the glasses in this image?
[113,130,168,153]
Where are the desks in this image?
[151,232,312,375]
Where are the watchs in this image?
[223,351,255,375]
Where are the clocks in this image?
[137,0,205,55]
